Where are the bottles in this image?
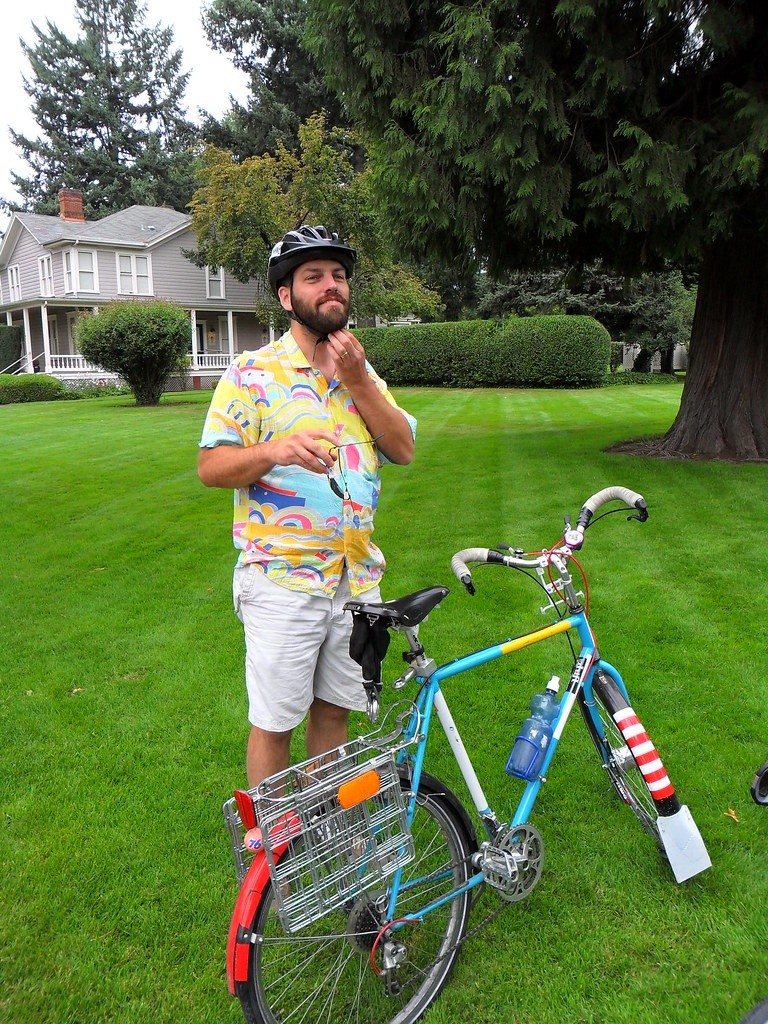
[506,675,560,780]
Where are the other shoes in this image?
[268,878,291,911]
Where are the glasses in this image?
[325,432,385,501]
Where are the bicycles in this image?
[224,483,712,1023]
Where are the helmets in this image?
[267,225,357,301]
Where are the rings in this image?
[341,351,348,358]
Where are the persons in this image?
[197,226,418,855]
